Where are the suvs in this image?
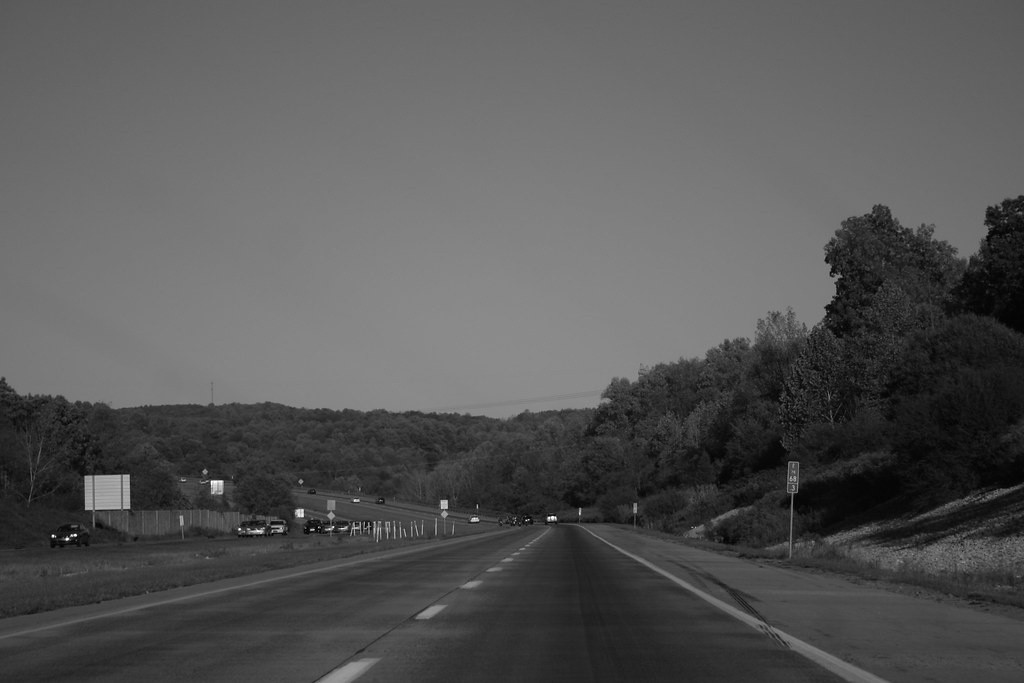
[375,497,385,504]
[352,497,360,503]
[237,518,289,538]
[468,514,480,524]
[498,513,558,526]
[307,488,316,495]
[177,477,187,483]
[198,478,209,485]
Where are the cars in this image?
[49,522,91,549]
[303,519,375,535]
